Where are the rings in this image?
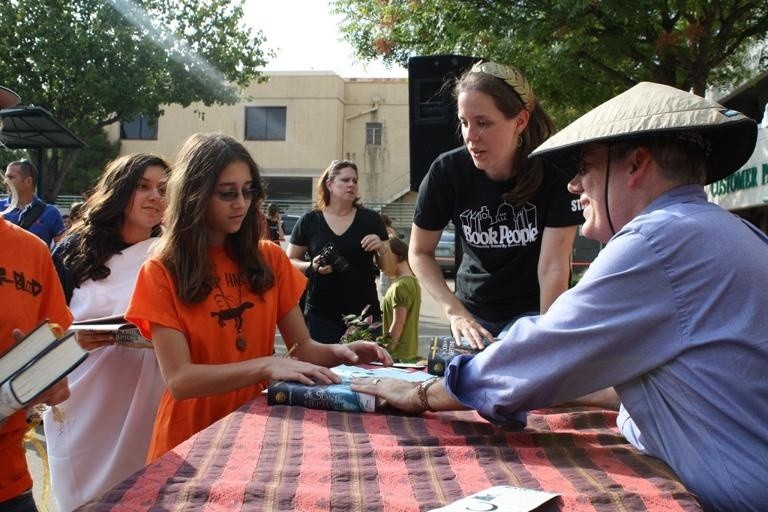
[371,377,380,384]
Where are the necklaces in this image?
[212,268,247,352]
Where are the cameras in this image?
[316,239,354,276]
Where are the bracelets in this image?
[417,373,440,413]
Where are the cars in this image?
[275,214,311,260]
[433,228,456,276]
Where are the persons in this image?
[1,211,75,512]
[265,203,285,245]
[408,59,583,350]
[375,237,422,362]
[0,158,95,255]
[42,151,173,512]
[379,213,397,295]
[350,81,767,511]
[285,159,397,343]
[122,131,395,467]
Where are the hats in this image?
[528,81,757,185]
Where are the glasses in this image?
[211,189,258,201]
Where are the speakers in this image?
[408,53,490,192]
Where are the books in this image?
[427,335,495,375]
[267,363,442,415]
[429,484,565,511]
[0,314,153,420]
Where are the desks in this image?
[64,359,707,511]
